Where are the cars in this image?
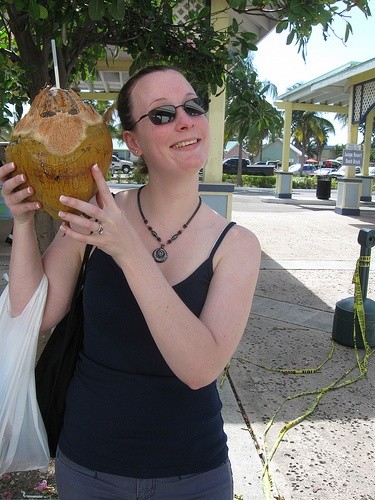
[356,167,375,177]
[309,167,337,177]
[329,165,362,180]
[254,161,283,170]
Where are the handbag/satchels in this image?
[35,193,116,458]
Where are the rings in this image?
[96,224,102,234]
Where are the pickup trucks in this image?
[222,157,274,176]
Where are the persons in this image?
[321,161,342,168]
[0,65,261,500]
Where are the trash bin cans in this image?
[316,175,331,199]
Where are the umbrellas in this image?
[306,159,317,162]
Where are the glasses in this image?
[129,98,211,130]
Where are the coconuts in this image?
[4,84,113,220]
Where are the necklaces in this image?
[138,186,202,262]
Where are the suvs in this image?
[104,155,135,174]
[289,163,317,176]
